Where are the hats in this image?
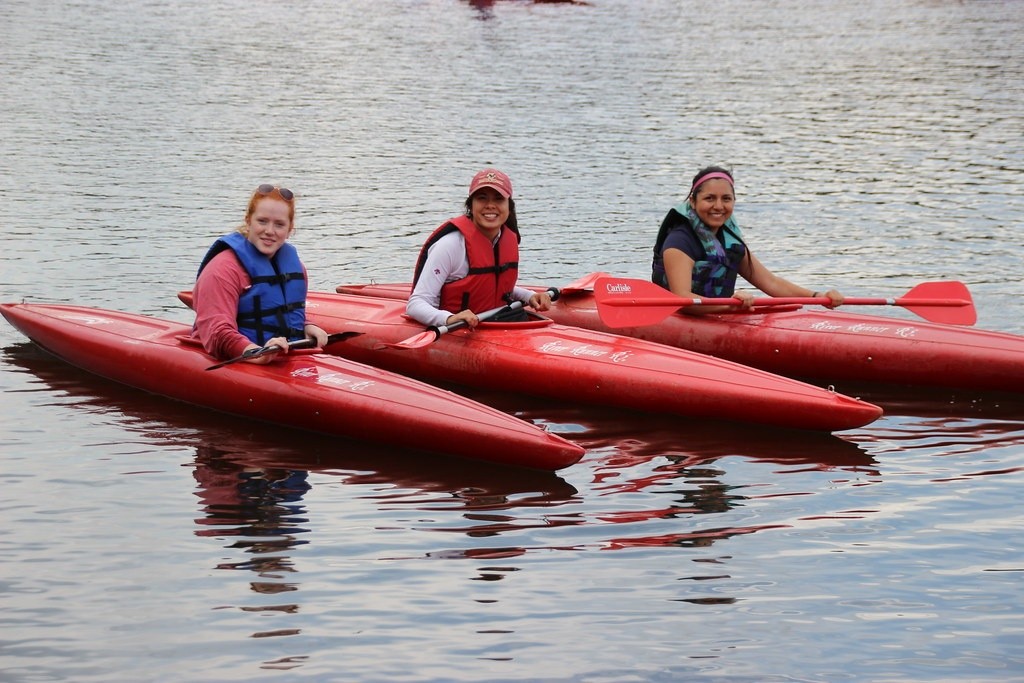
[466,170,514,202]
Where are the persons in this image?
[404,167,552,333]
[188,184,330,362]
[651,164,846,318]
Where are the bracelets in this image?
[811,291,819,298]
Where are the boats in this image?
[177,292,885,434]
[0,302,587,472]
[336,282,1024,399]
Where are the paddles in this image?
[204,331,367,371]
[593,277,976,329]
[383,272,608,349]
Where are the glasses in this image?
[252,184,295,206]
